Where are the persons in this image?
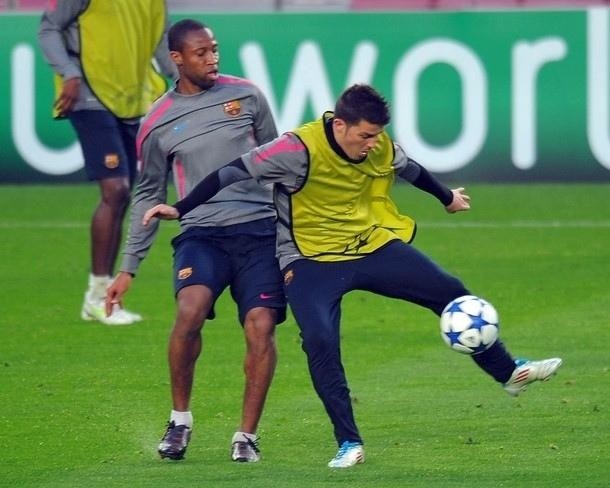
[36,0,184,325]
[104,15,283,462]
[141,83,563,469]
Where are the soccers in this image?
[439,295,499,356]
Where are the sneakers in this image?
[328,441,365,467]
[158,421,192,460]
[503,358,562,396]
[81,300,142,326]
[232,434,260,462]
